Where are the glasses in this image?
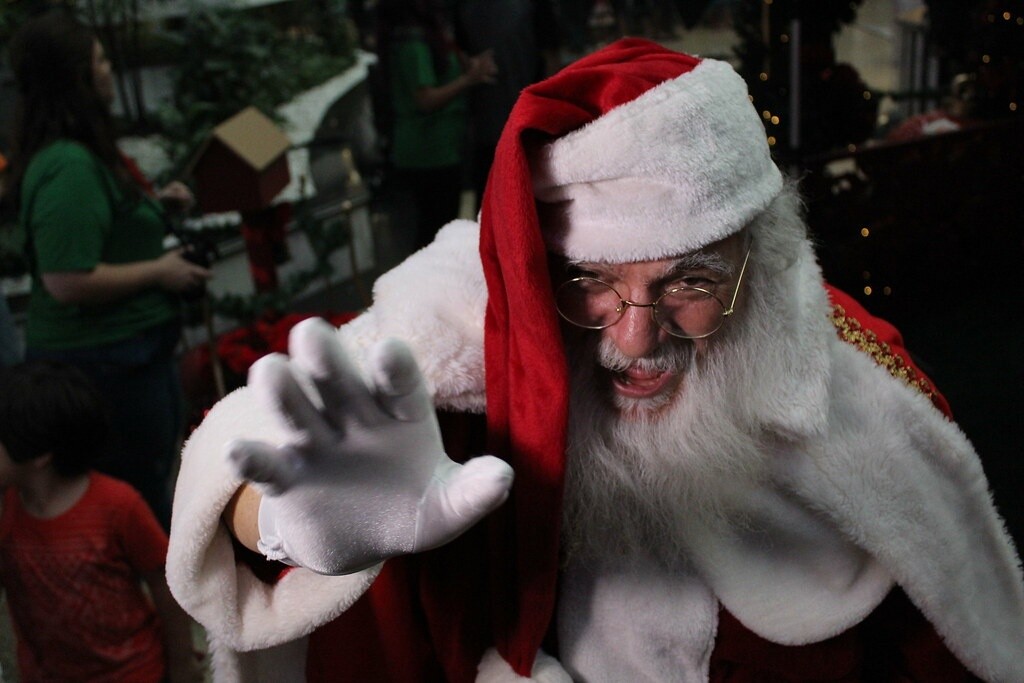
[553,234,758,340]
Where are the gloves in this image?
[229,316,515,575]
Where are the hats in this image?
[477,39,786,683]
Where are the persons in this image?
[381,12,498,255]
[10,15,214,536]
[0,362,197,683]
[564,0,623,59]
[164,36,1024,682]
